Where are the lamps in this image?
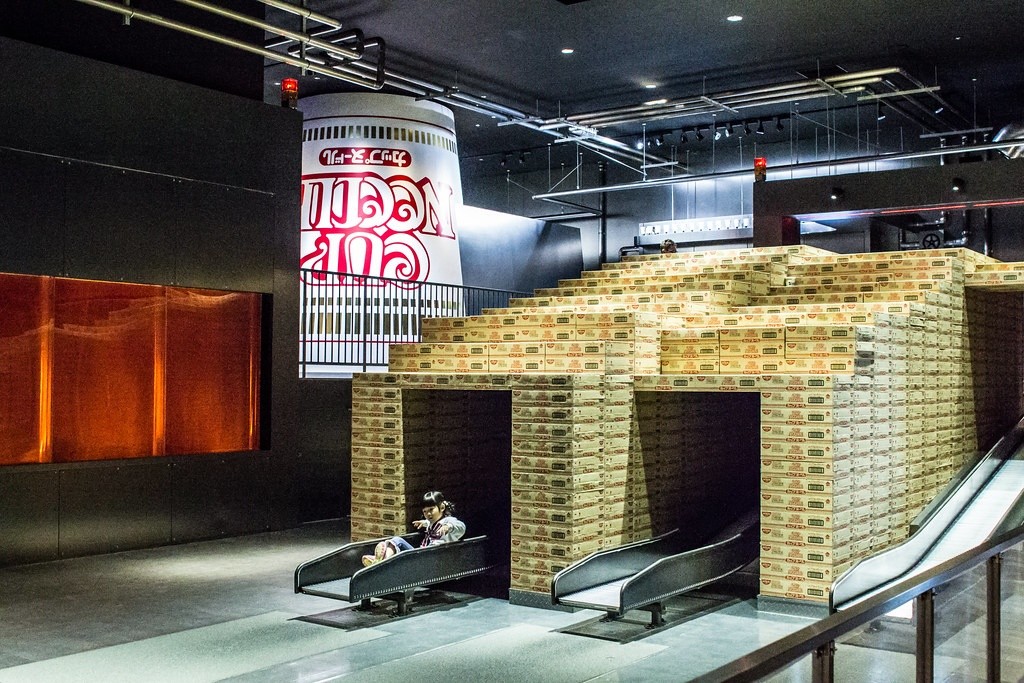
[755,158,767,180]
[281,78,297,109]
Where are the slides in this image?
[293,498,511,616]
[552,492,760,627]
[831,416,1023,622]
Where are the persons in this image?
[361,490,466,567]
[659,239,678,253]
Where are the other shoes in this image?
[362,555,376,567]
[375,542,388,561]
